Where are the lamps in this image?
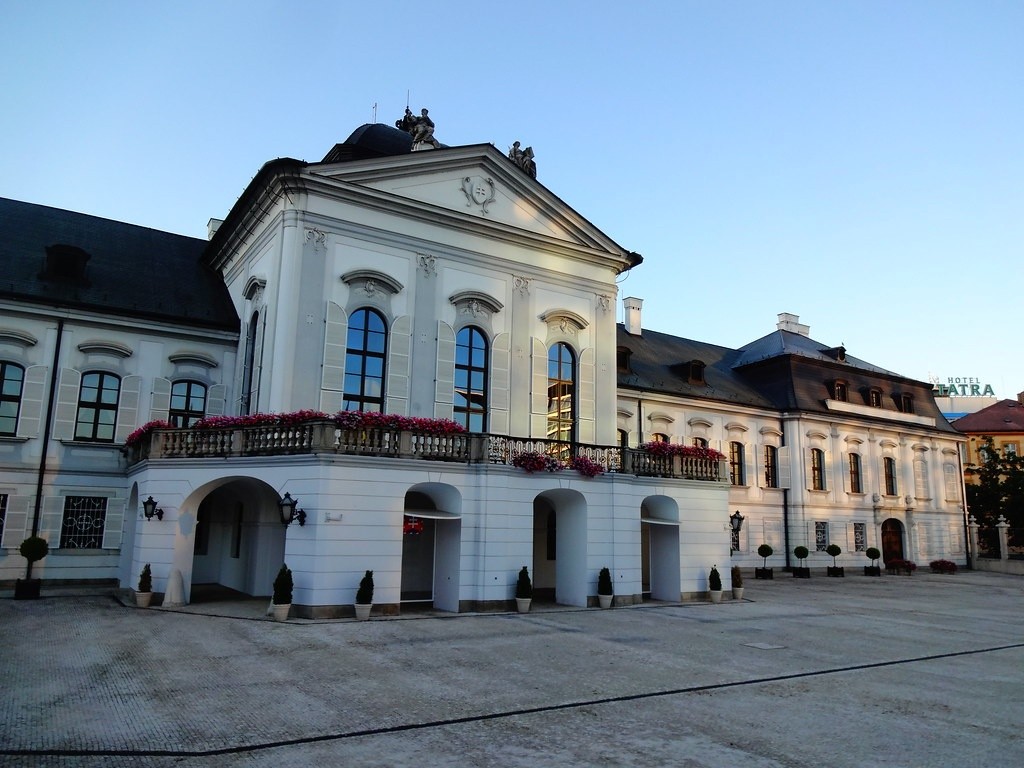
[277,491,307,528]
[142,495,164,521]
[729,509,745,531]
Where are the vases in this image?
[888,566,911,575]
[932,568,953,574]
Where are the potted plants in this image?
[597,566,613,608]
[864,547,880,575]
[826,543,844,576]
[793,545,811,577]
[731,564,744,598]
[514,564,533,613]
[272,563,294,622]
[13,536,49,601]
[354,568,374,619]
[754,544,773,579]
[710,566,723,603]
[134,562,154,606]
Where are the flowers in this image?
[571,455,604,479]
[125,417,174,446]
[884,558,917,573]
[543,455,565,472]
[332,407,467,434]
[192,408,328,428]
[643,439,727,459]
[929,559,957,571]
[512,450,544,474]
